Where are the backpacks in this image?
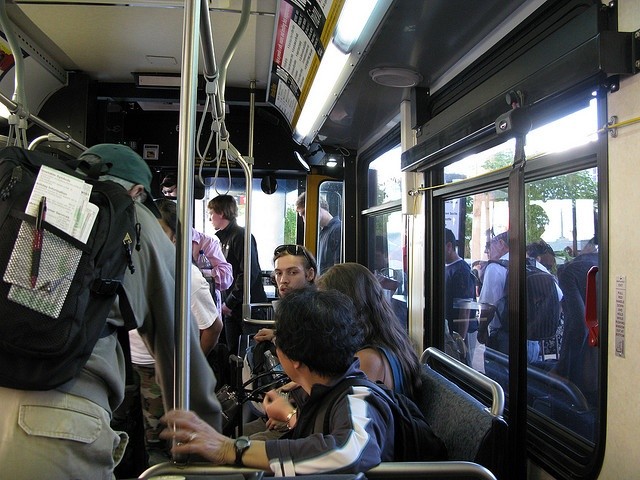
[488,258,559,341]
[312,377,449,462]
[0,146,141,390]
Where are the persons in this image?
[159,286,394,477]
[247,328,276,389]
[315,263,423,413]
[273,244,316,300]
[525,238,556,270]
[127,198,223,466]
[472,261,487,284]
[478,226,563,393]
[0,143,222,480]
[374,235,407,331]
[546,204,599,409]
[208,195,275,390]
[444,228,479,368]
[296,191,341,276]
[160,175,178,198]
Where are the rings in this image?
[189,432,196,441]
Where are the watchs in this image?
[234,435,250,467]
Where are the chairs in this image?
[385,345,505,461]
[456,343,603,443]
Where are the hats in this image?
[75,144,163,219]
[160,175,177,187]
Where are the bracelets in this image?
[286,409,297,429]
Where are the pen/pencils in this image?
[32,196,47,288]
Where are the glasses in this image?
[274,245,313,266]
[164,187,177,194]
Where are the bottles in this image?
[263,351,283,378]
[197,250,214,293]
